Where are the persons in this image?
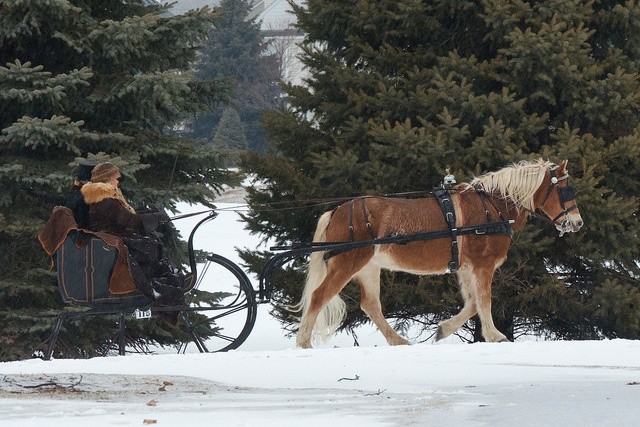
[65,163,96,229]
[80,162,169,284]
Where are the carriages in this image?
[38,156,585,361]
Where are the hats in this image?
[91,162,120,183]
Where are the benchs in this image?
[38,206,155,308]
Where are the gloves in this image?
[157,210,171,223]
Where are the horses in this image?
[279,157,585,350]
